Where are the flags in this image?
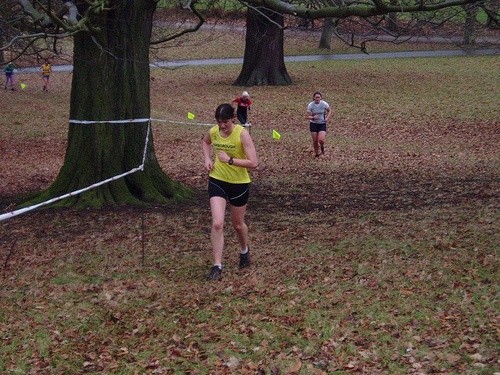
[273,130,281,140]
[188,112,195,119]
[21,83,27,90]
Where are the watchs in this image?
[229,157,233,165]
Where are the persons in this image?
[40,60,51,91]
[202,104,257,280]
[306,92,331,157]
[4,62,15,90]
[232,91,253,126]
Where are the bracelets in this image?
[312,116,314,120]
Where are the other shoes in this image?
[239,246,251,269]
[206,264,223,282]
[242,92,249,97]
